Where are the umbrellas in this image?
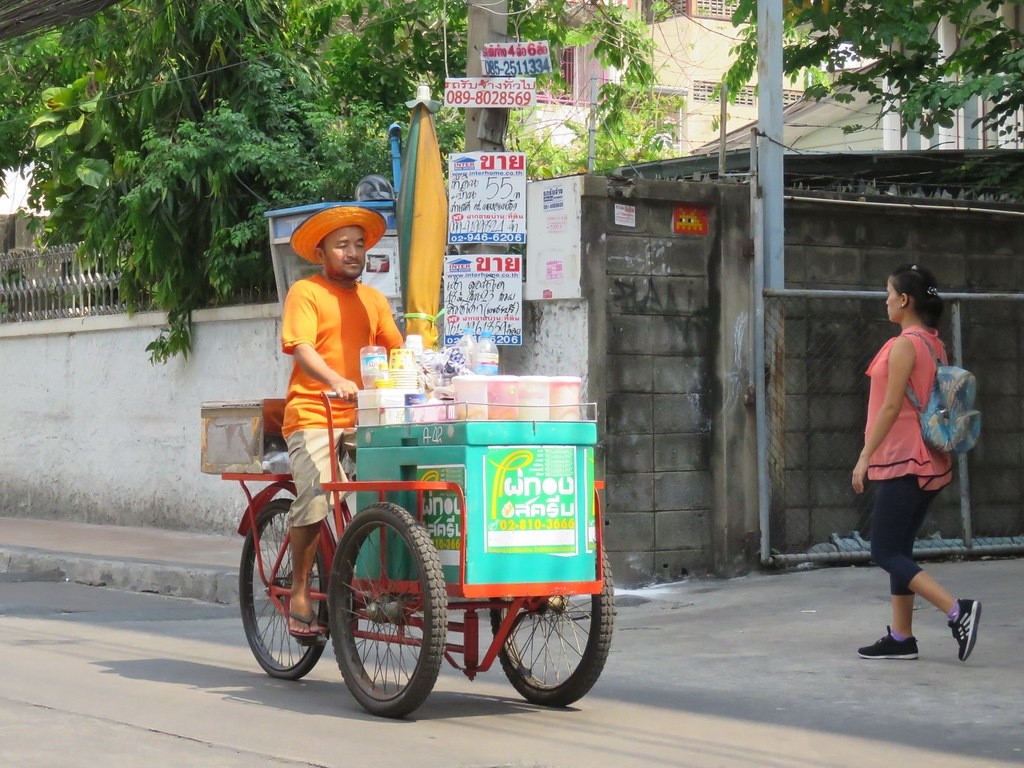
[395,87,449,355]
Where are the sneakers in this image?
[856,622,919,661]
[947,599,982,661]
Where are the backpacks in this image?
[900,332,980,456]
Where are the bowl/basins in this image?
[388,347,419,389]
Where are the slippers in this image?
[287,608,321,637]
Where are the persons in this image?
[281,206,406,637]
[852,264,982,662]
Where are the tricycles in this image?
[219,390,621,721]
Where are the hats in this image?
[290,206,386,265]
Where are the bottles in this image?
[359,346,387,389]
[473,330,499,376]
[375,361,394,390]
[261,451,290,474]
[455,328,476,369]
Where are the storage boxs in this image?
[200,399,287,474]
[357,389,424,426]
